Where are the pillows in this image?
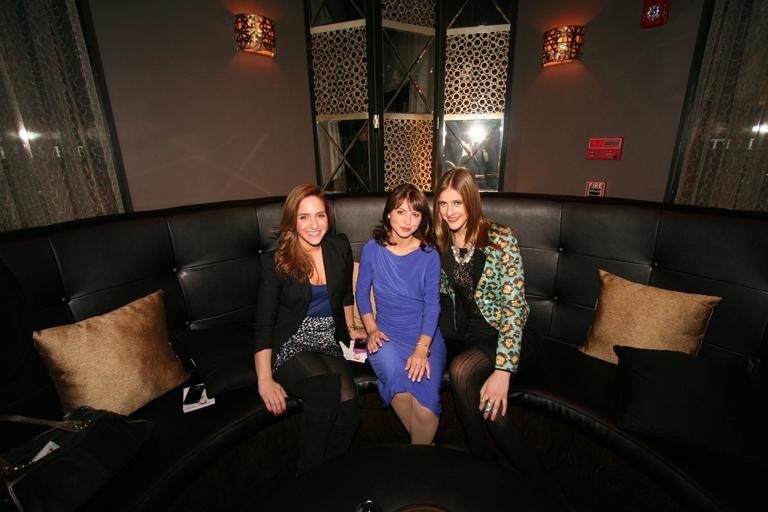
[32,289,259,415]
[579,263,721,445]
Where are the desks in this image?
[284,445,540,512]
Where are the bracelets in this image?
[415,341,431,358]
[346,323,356,333]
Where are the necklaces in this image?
[451,239,476,266]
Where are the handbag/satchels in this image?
[0,408,157,511]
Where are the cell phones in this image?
[353,338,368,353]
[183,385,204,404]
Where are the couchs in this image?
[1,191,767,512]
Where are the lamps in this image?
[234,13,277,59]
[542,24,584,68]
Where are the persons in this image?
[354,183,449,446]
[249,182,369,485]
[434,166,533,467]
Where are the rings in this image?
[479,397,485,404]
[416,363,422,368]
[484,400,493,412]
